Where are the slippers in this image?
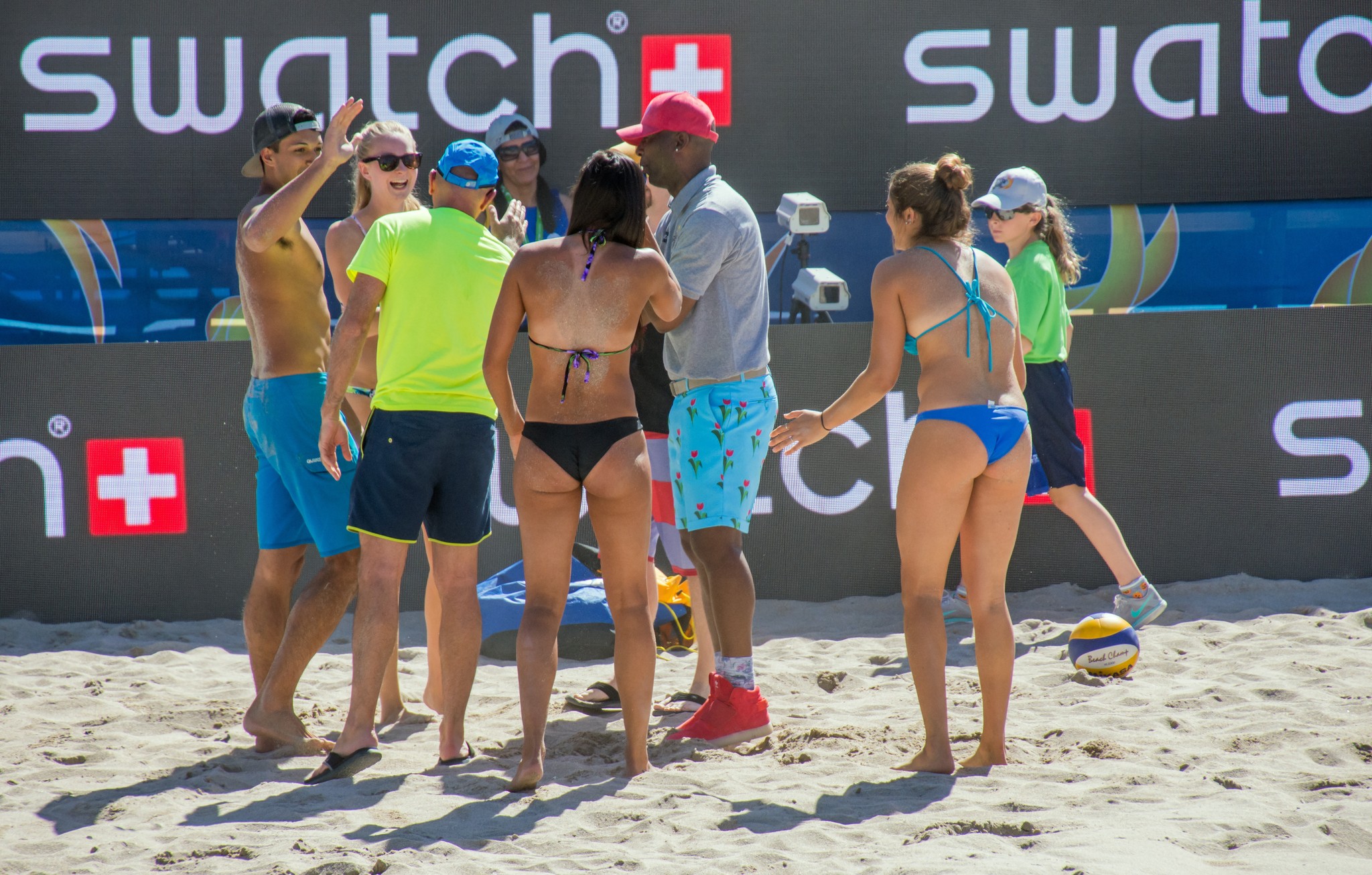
[652,690,707,717]
[439,740,476,765]
[564,681,621,710]
[303,746,382,784]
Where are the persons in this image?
[942,167,1169,629]
[228,95,778,792]
[768,155,1032,775]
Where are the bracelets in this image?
[820,412,832,430]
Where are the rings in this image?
[790,438,794,442]
[785,422,789,431]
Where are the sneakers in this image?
[1111,584,1168,632]
[665,671,774,746]
[941,589,974,626]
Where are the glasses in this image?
[362,153,423,172]
[984,206,1029,221]
[496,138,540,162]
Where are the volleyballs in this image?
[1068,612,1140,679]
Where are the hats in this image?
[484,114,548,170]
[241,103,320,178]
[437,138,499,189]
[616,90,719,143]
[971,166,1047,210]
